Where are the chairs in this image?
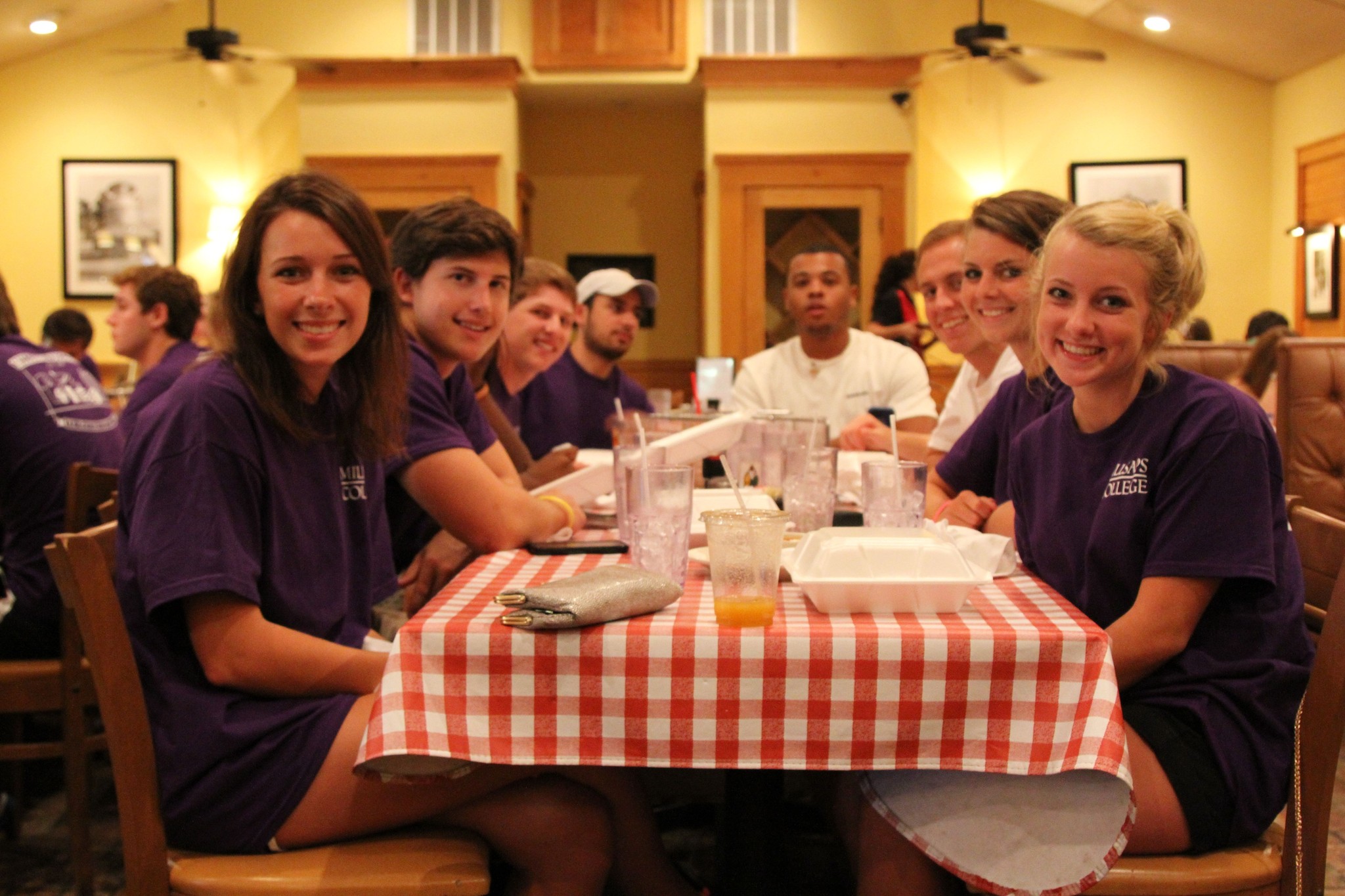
[1,460,127,893]
[1085,490,1344,896]
[38,517,492,896]
[1278,337,1343,633]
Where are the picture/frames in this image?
[60,157,177,300]
[1068,159,1189,214]
[1302,222,1340,319]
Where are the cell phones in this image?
[524,540,629,555]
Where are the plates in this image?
[687,545,792,581]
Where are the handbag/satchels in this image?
[495,565,681,632]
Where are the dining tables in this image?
[344,454,1136,893]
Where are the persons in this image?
[115,174,603,896]
[107,264,209,517]
[518,268,657,452]
[0,276,119,658]
[873,189,1297,896]
[724,243,942,468]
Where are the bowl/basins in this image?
[792,524,993,615]
[688,485,780,547]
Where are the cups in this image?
[612,445,666,544]
[609,410,656,449]
[861,458,929,529]
[623,466,694,586]
[696,507,788,630]
[778,444,838,531]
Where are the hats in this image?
[576,269,659,311]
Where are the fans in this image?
[97,0,337,89]
[891,0,1106,88]
